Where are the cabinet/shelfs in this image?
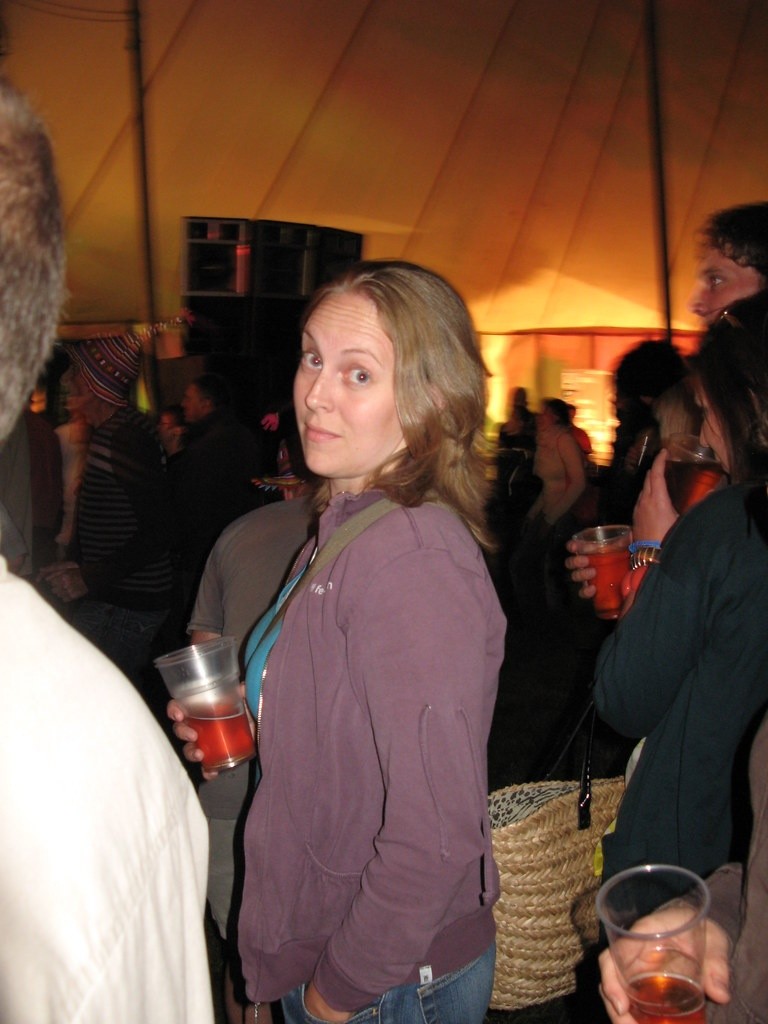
[180,216,365,430]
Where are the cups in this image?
[633,432,658,469]
[663,433,723,516]
[153,633,256,776]
[573,524,631,618]
[595,865,710,1024]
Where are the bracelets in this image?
[628,540,661,553]
[630,547,660,570]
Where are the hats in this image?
[62,308,197,407]
[616,342,688,396]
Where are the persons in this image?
[476,199,768,1024]
[0,77,215,1024]
[168,261,509,1024]
[0,317,325,1024]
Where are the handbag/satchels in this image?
[570,486,600,520]
[486,696,631,1010]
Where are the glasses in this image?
[714,304,749,334]
